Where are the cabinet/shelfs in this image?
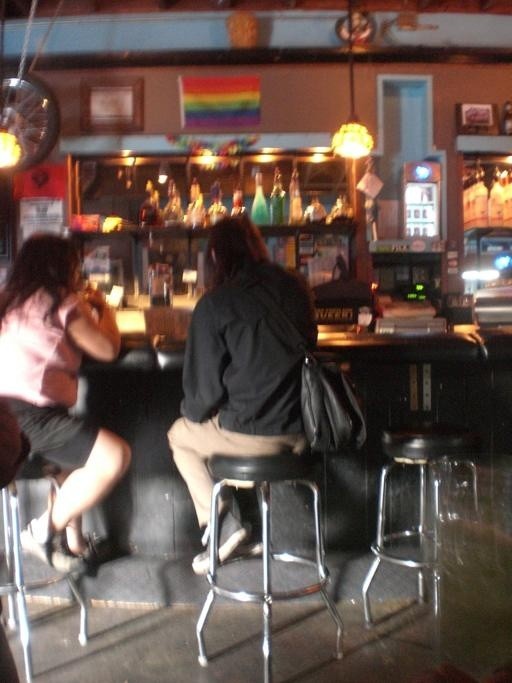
[66,150,373,317]
[451,136,512,324]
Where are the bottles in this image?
[205,181,225,226]
[289,169,303,226]
[150,264,173,306]
[185,178,205,227]
[251,172,268,224]
[423,228,428,236]
[161,179,179,226]
[140,180,158,226]
[415,228,420,236]
[462,167,512,228]
[406,209,411,217]
[326,193,353,225]
[414,210,420,218]
[407,228,410,237]
[503,100,512,136]
[231,190,247,215]
[422,209,427,218]
[270,168,285,225]
[303,190,326,224]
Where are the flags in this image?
[177,76,262,130]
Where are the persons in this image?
[0,232,133,565]
[167,208,318,576]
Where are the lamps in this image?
[0,0,21,168]
[332,0,374,159]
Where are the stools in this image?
[196,452,345,683]
[362,425,494,629]
[0,461,87,683]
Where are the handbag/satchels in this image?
[235,273,367,454]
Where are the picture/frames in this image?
[456,101,498,128]
[78,73,144,133]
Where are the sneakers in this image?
[20,520,87,574]
[235,531,261,557]
[80,535,116,566]
[191,508,246,575]
